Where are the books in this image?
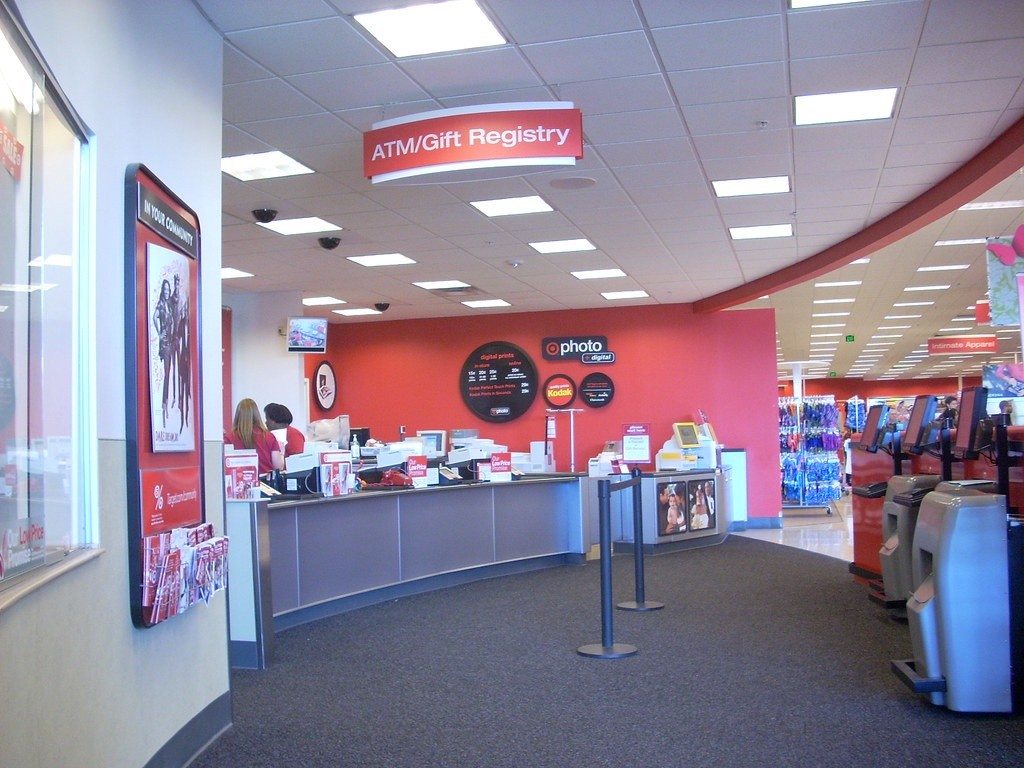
[139,522,229,624]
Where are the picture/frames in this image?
[460,341,539,424]
[656,481,687,537]
[313,361,337,411]
[687,479,715,532]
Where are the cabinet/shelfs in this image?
[140,521,230,624]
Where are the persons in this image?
[876,396,1014,448]
[657,480,715,536]
[263,403,305,457]
[224,398,284,494]
[152,273,190,434]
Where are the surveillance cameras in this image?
[318,237,341,251]
[252,209,277,223]
[375,303,390,311]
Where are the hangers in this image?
[780,450,840,464]
[778,394,837,409]
[778,425,842,438]
[781,478,841,490]
[847,396,864,404]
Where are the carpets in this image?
[184,535,1024,768]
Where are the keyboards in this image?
[894,487,934,501]
[852,481,889,496]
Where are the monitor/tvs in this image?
[902,395,938,455]
[672,422,701,448]
[858,405,888,453]
[417,430,447,457]
[286,316,327,352]
[954,388,988,460]
